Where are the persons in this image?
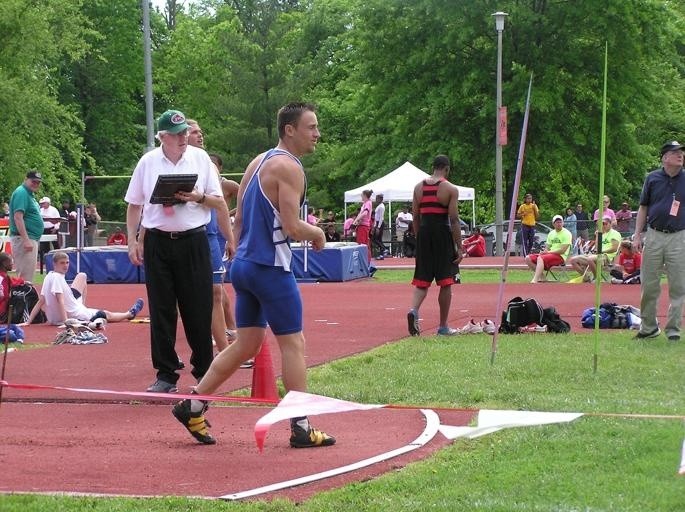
[408,154,464,337]
[171,103,336,448]
[516,193,642,285]
[122,112,236,393]
[462,226,487,257]
[173,118,254,369]
[0,171,145,330]
[632,142,685,340]
[304,186,411,264]
[207,152,240,342]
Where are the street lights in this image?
[492,9,511,256]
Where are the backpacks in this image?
[498,296,545,336]
[8,284,48,324]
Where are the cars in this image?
[478,219,554,256]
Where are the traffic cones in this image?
[248,331,282,401]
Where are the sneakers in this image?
[481,318,495,335]
[518,323,547,333]
[238,360,254,369]
[407,309,421,337]
[668,335,681,341]
[437,326,462,336]
[460,319,483,335]
[171,390,217,445]
[146,379,179,394]
[127,298,145,320]
[376,249,389,260]
[631,327,661,340]
[226,329,237,341]
[289,415,336,449]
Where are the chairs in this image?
[542,245,571,283]
[583,246,618,283]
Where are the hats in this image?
[552,214,563,224]
[39,196,51,204]
[660,140,685,160]
[26,170,43,182]
[156,110,192,136]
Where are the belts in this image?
[650,224,685,234]
